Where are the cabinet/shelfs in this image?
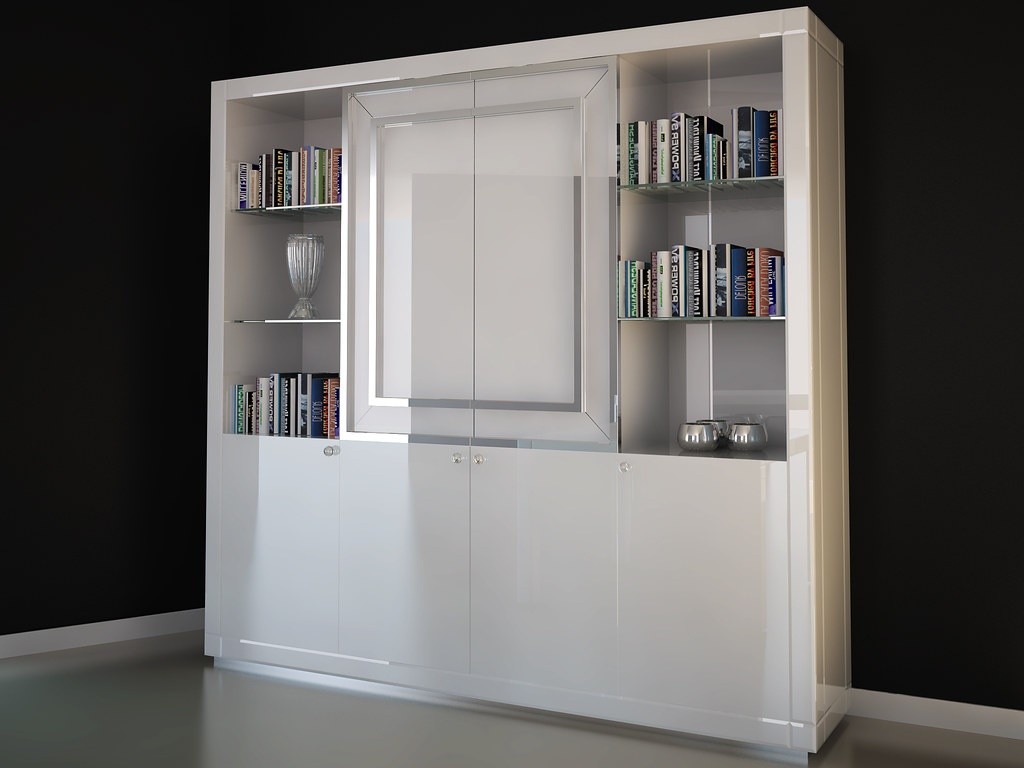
[194,6,852,768]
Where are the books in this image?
[235,372,339,439]
[622,244,784,318]
[237,145,341,208]
[627,105,784,185]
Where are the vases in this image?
[286,234,326,320]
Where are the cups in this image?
[677,414,769,451]
